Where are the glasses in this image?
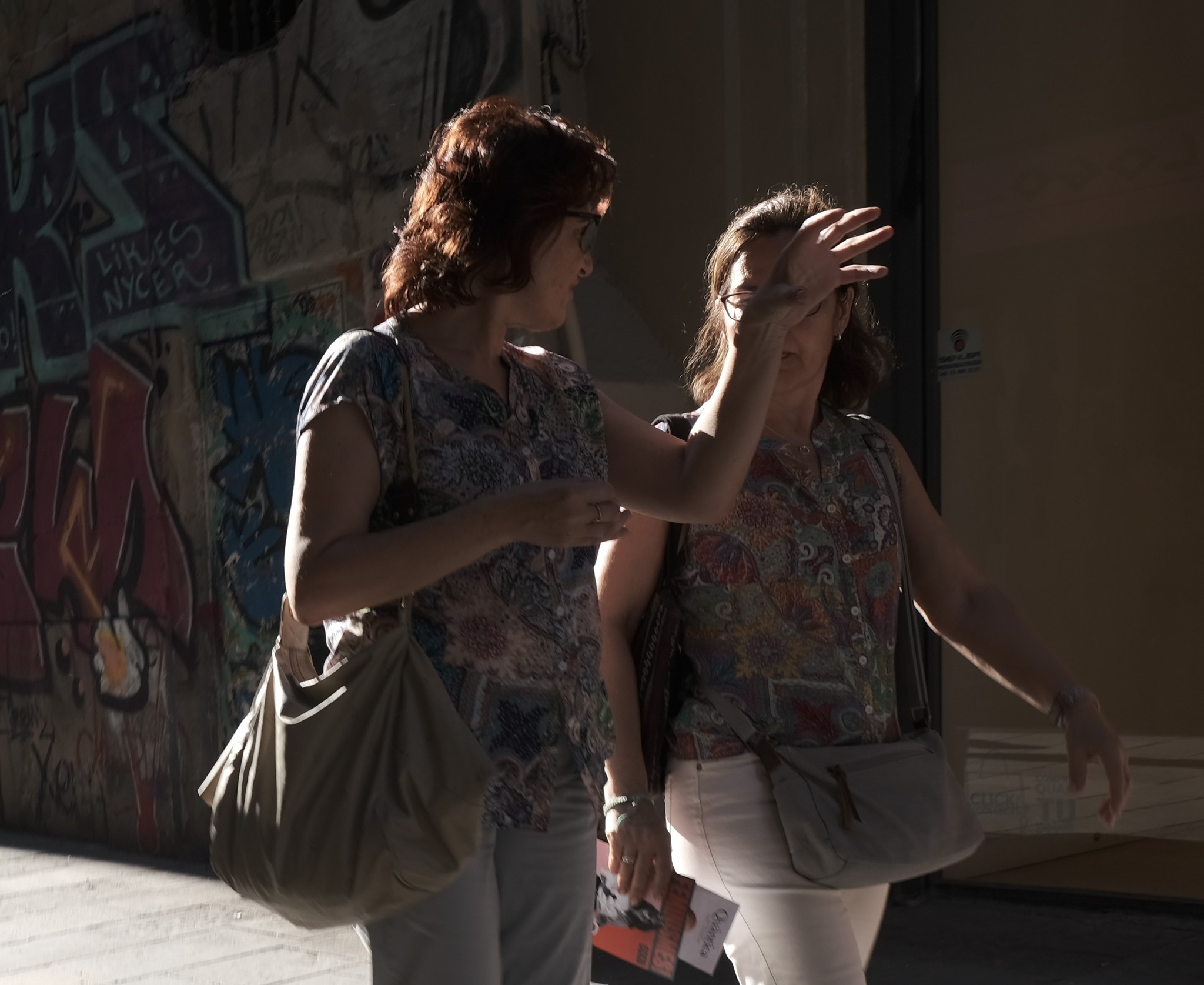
[722,291,823,321]
[564,212,608,254]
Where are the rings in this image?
[619,854,635,865]
[595,505,603,523]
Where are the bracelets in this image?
[1046,682,1101,726]
[601,793,658,827]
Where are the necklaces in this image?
[765,424,813,455]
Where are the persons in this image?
[279,95,897,985]
[590,184,1135,985]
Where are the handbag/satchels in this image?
[767,730,988,889]
[199,329,494,929]
